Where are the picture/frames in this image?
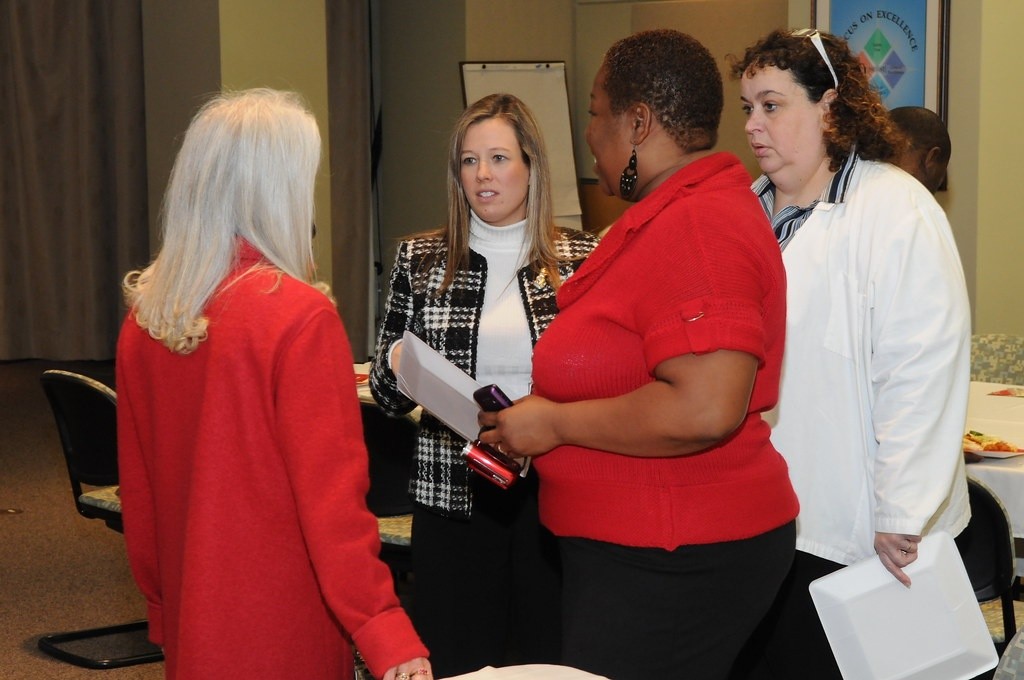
[811,0,950,191]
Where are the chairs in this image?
[39,370,164,668]
[954,477,1017,680]
[971,333,1024,386]
[362,402,419,573]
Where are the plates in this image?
[809,533,999,680]
[964,445,1023,458]
[395,330,530,477]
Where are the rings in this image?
[498,444,506,453]
[906,543,912,549]
[395,672,410,680]
[410,668,428,677]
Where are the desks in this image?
[354,361,1024,578]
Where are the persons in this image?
[116,88,435,680]
[885,105,951,196]
[370,92,602,680]
[725,24,973,680]
[477,27,800,680]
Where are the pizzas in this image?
[963,431,1024,453]
[987,386,1024,397]
